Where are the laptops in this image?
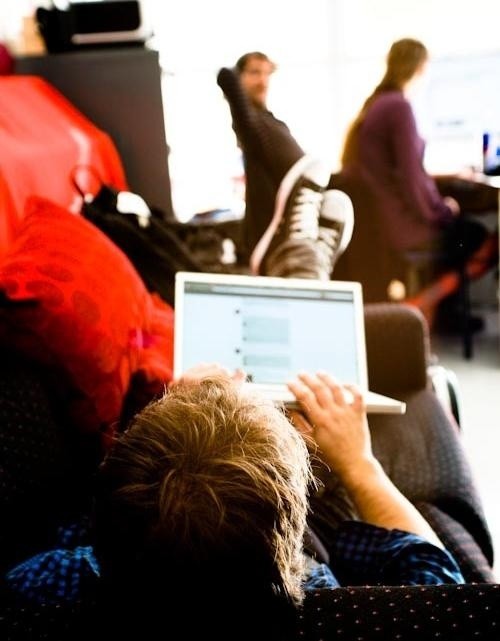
[171,271,407,415]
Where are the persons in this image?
[1,148,467,620]
[215,50,329,242]
[340,38,484,338]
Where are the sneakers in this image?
[247,149,330,275]
[317,188,354,271]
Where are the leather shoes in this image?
[435,314,482,339]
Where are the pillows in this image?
[0,186,174,435]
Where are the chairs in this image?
[218,63,297,246]
